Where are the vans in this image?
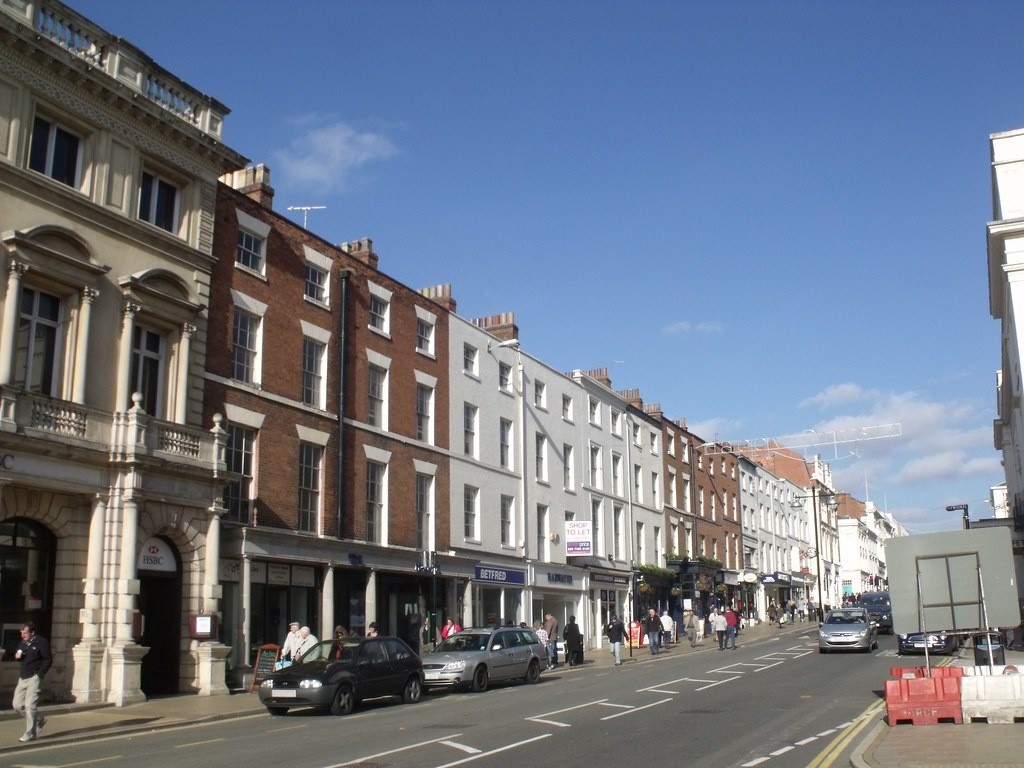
[858,592,895,636]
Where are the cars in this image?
[816,607,879,653]
[896,630,964,656]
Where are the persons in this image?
[280,622,300,662]
[684,610,700,647]
[709,605,738,650]
[563,615,582,665]
[607,615,628,665]
[660,610,673,648]
[330,624,350,660]
[768,598,814,629]
[519,622,526,626]
[419,617,442,655]
[367,622,381,637]
[645,609,664,655]
[534,611,559,668]
[12,620,53,741]
[295,626,320,663]
[443,618,460,639]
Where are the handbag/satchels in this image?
[779,618,788,625]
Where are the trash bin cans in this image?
[972,631,1006,666]
[809,608,816,622]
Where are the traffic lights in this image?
[868,575,874,586]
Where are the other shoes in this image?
[18,732,38,742]
[35,718,47,737]
[650,652,656,656]
[725,646,730,650]
[720,648,724,650]
[732,647,735,651]
[569,663,577,667]
[691,644,695,648]
[549,664,556,670]
[667,645,670,649]
[615,663,620,666]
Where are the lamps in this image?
[608,554,613,561]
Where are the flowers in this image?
[694,577,708,591]
[716,583,728,593]
[634,581,653,595]
[671,587,683,598]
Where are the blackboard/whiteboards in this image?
[661,621,678,642]
[250,643,279,689]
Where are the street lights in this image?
[790,485,841,629]
[945,503,970,531]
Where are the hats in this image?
[288,622,300,626]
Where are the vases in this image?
[696,584,706,591]
[640,586,649,593]
[672,591,680,597]
[718,588,725,592]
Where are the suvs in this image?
[419,624,550,694]
[257,635,425,716]
